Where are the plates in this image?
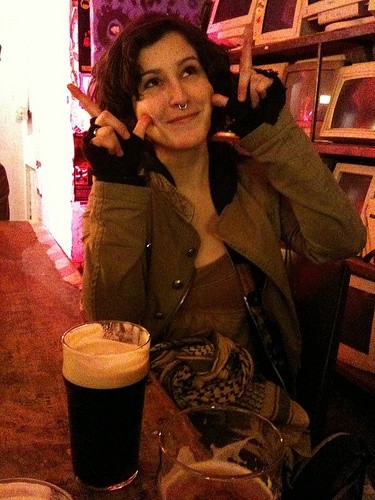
[0,477,73,500]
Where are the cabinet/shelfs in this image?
[211,20,375,400]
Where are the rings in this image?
[93,127,99,137]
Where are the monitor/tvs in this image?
[206,0,375,374]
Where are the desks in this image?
[0,219,213,500]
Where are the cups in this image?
[61,320,150,491]
[155,404,285,500]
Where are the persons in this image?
[67,12,367,500]
